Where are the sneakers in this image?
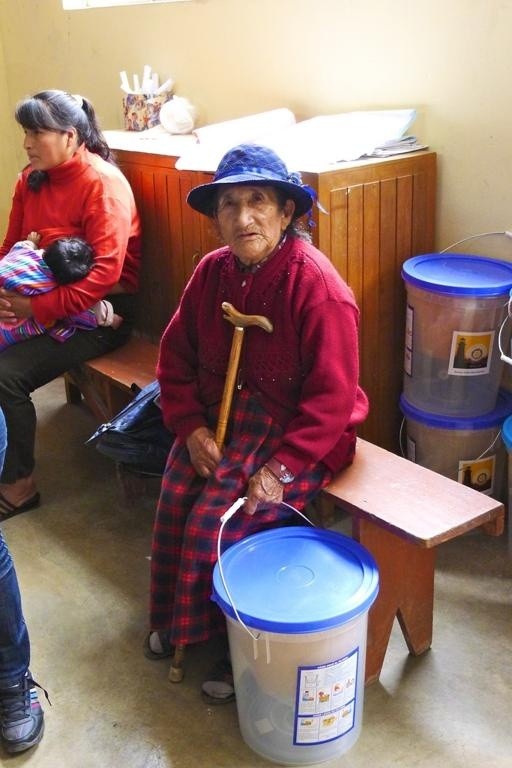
[0,668,46,754]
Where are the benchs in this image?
[62,331,503,685]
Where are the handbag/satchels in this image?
[85,380,175,474]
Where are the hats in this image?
[187,146,313,220]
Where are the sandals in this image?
[200,651,235,705]
[143,631,194,660]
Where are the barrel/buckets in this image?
[211,495,378,765]
[397,388,512,500]
[401,232,512,417]
[502,415,512,457]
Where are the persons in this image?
[0,230,124,355]
[0,89,144,523]
[0,408,45,756]
[142,144,372,705]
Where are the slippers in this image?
[0,492,40,522]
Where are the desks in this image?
[101,128,437,455]
